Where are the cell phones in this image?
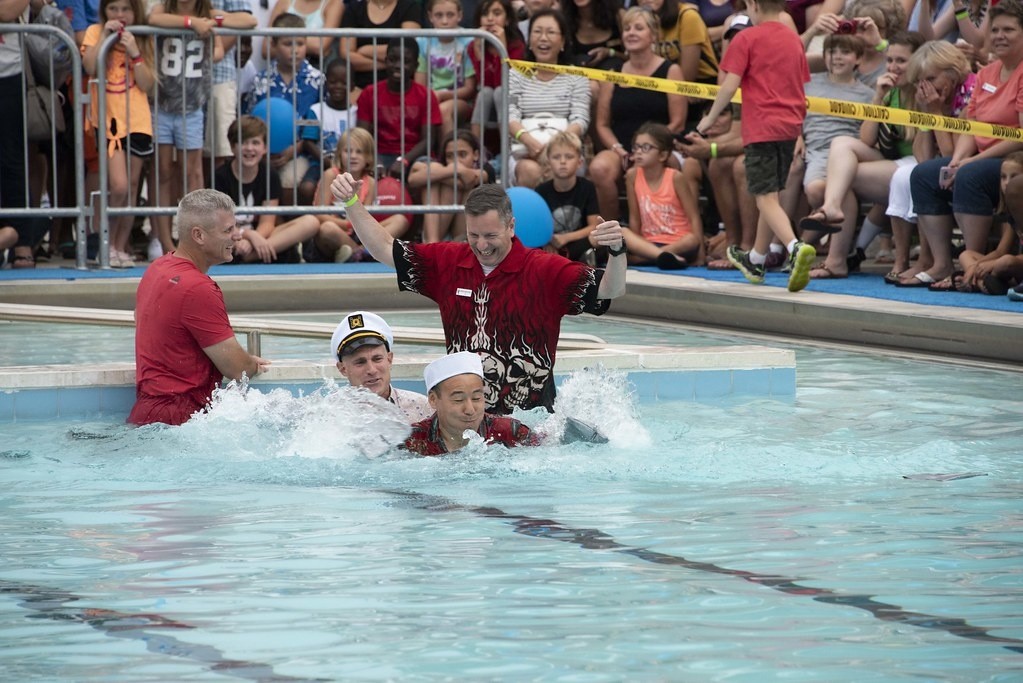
[939,167,957,190]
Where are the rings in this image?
[926,94,929,98]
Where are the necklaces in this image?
[372,0,393,10]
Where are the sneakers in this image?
[1007,282,1023,301]
[95,248,136,268]
[786,241,817,291]
[726,245,767,282]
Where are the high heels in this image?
[11,242,36,269]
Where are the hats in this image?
[423,351,485,395]
[724,13,753,40]
[329,310,393,362]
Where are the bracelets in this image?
[610,49,616,59]
[130,51,142,60]
[874,39,889,52]
[187,16,192,29]
[955,11,969,20]
[954,6,967,14]
[376,164,384,168]
[611,143,623,152]
[515,129,527,140]
[711,142,717,159]
[605,239,627,258]
[184,15,188,29]
[128,53,144,64]
[918,127,930,133]
[341,193,358,207]
[396,157,409,166]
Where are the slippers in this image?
[800,210,842,233]
[980,273,1010,296]
[808,262,848,278]
[896,271,953,291]
[952,270,976,292]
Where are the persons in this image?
[124,190,271,427]
[331,169,629,413]
[396,351,610,459]
[330,311,427,407]
[1,0,1023,299]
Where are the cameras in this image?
[833,21,857,34]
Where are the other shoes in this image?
[655,251,688,270]
[760,251,784,267]
[221,248,243,264]
[707,253,736,270]
[333,244,363,263]
[146,237,164,262]
[885,267,911,283]
[276,246,301,264]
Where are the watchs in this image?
[214,14,223,27]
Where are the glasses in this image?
[630,142,663,155]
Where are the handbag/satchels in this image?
[509,110,570,159]
[20,3,78,89]
[874,86,902,159]
[24,84,68,140]
